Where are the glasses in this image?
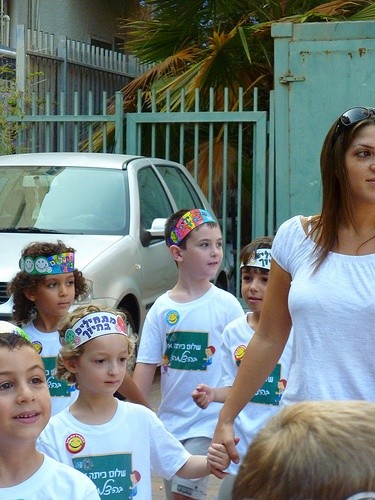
[331,107,375,147]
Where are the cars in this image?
[0,151,231,372]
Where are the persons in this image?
[8,240,152,417]
[34,304,231,500]
[128,208,245,500]
[231,399,375,500]
[0,320,101,500]
[191,237,294,500]
[206,106,375,479]
